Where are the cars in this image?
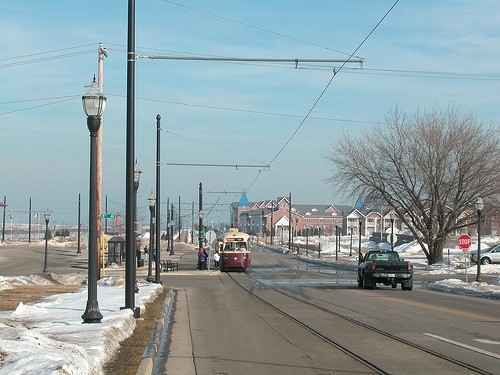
[471,242,500,265]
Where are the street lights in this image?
[42,209,51,273]
[80,72,108,324]
[305,222,310,256]
[474,197,485,282]
[335,219,339,262]
[133,158,143,293]
[198,209,205,248]
[146,190,157,283]
[389,211,397,251]
[358,215,364,261]
[318,223,322,258]
[166,222,171,252]
[168,220,176,256]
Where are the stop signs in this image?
[457,234,472,249]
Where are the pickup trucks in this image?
[357,249,414,291]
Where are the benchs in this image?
[160,259,179,273]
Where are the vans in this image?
[213,228,252,272]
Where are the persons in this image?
[197,247,208,270]
[144,245,148,262]
